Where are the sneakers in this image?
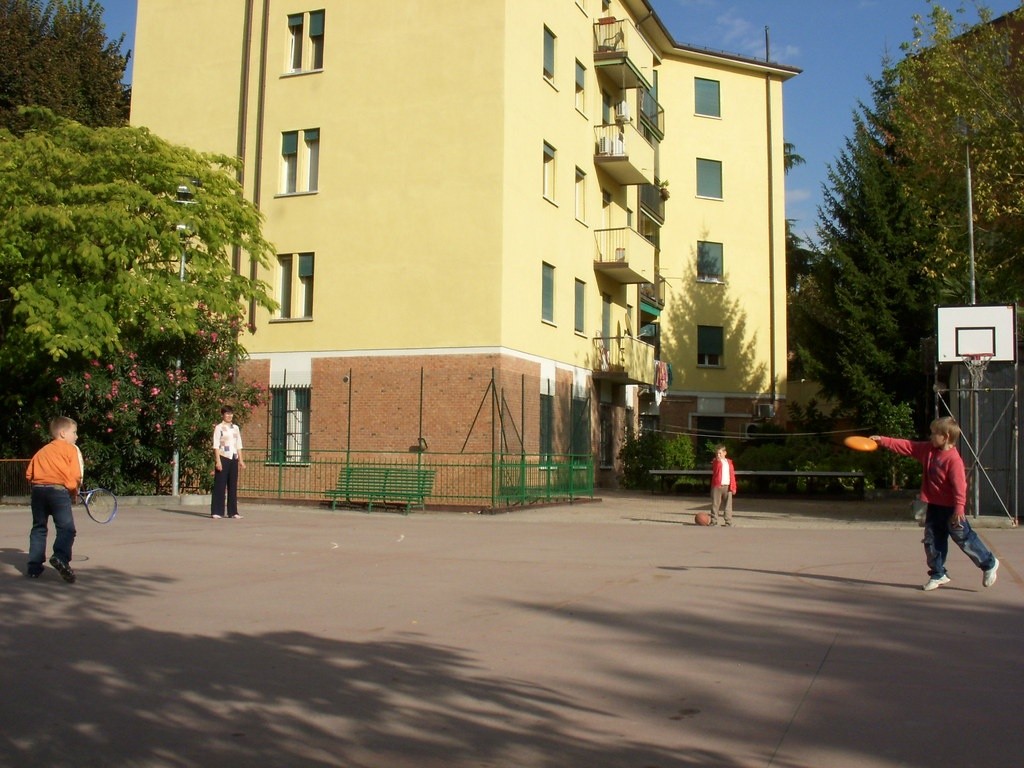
[982,556,1000,587]
[922,573,951,591]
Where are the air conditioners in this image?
[615,98,631,123]
[599,138,614,154]
[754,404,774,418]
[739,423,763,440]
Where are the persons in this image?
[211,405,246,519]
[869,416,999,591]
[26,416,84,584]
[709,444,737,527]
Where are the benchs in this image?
[323,467,437,515]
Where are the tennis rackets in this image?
[77,488,118,524]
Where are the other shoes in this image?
[229,514,244,519]
[28,568,38,579]
[708,522,718,526]
[723,523,730,528]
[212,514,221,519]
[49,555,76,583]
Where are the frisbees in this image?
[845,436,877,451]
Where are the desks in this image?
[648,469,864,501]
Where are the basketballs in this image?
[695,511,710,526]
